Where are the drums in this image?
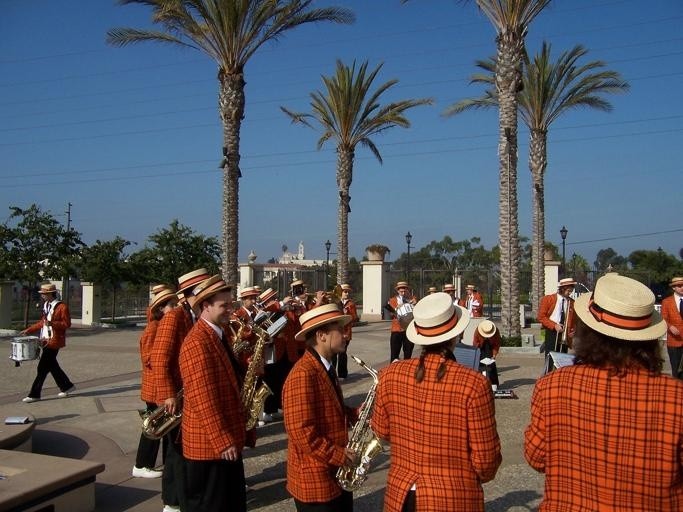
[396,304,415,328]
[10,334,40,362]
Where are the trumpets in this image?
[306,285,343,304]
[251,304,274,327]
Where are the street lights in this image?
[557,225,568,277]
[324,237,332,268]
[657,245,664,263]
[571,250,576,276]
[403,230,412,284]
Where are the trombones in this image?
[141,341,250,440]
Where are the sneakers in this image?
[53,383,78,398]
[130,463,168,480]
[20,393,43,406]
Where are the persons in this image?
[522,270,682,512]
[385,280,502,397]
[660,275,682,378]
[15,281,76,402]
[371,291,504,511]
[536,278,576,374]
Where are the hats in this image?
[393,281,476,296]
[557,276,578,288]
[293,303,353,342]
[148,268,354,313]
[571,270,671,342]
[668,276,683,287]
[475,319,499,340]
[405,291,473,348]
[36,283,63,296]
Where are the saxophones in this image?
[235,314,274,431]
[335,356,385,491]
